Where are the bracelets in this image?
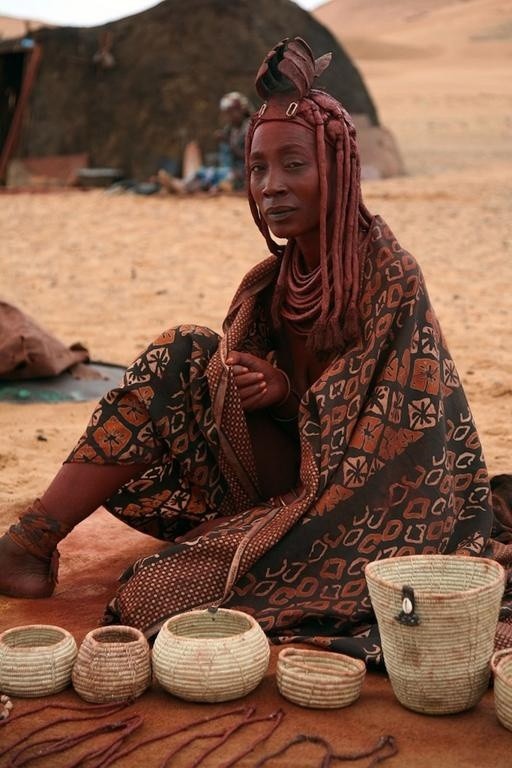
[277,367,292,408]
[275,390,303,425]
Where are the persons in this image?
[159,91,257,192]
[1,32,512,673]
